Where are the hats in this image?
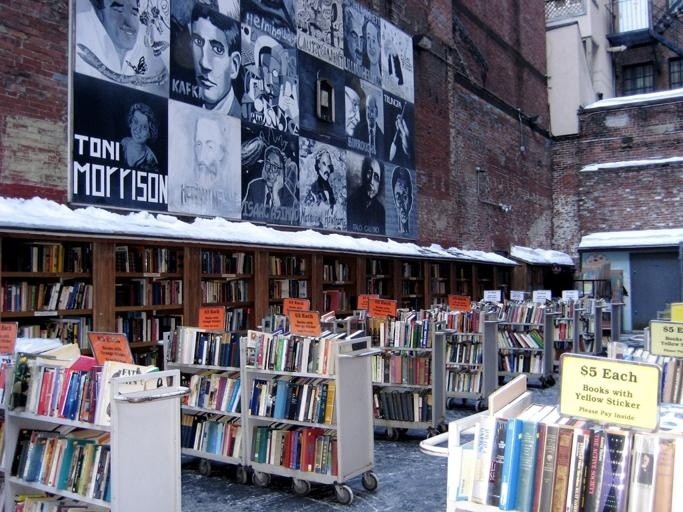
[344,69,365,99]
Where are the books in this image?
[1,241,683,512]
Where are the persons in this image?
[361,20,381,85]
[185,3,242,118]
[344,70,366,138]
[392,167,412,233]
[296,1,337,46]
[302,149,336,228]
[347,156,385,233]
[395,113,410,155]
[242,147,300,225]
[121,102,160,171]
[194,117,230,191]
[382,23,403,85]
[249,46,299,137]
[75,0,170,98]
[344,7,365,64]
[354,95,384,159]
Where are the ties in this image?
[265,191,271,214]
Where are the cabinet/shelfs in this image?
[0,227,682,512]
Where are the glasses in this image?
[265,161,282,172]
[345,91,361,106]
